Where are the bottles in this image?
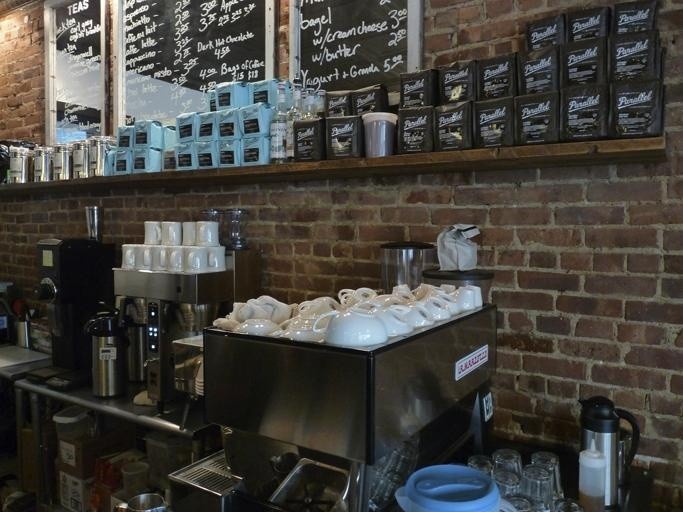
[266,84,328,162]
[576,440,609,510]
[6,135,116,184]
[83,301,129,399]
[577,396,642,493]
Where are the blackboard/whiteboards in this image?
[284,0,424,106]
[42,0,109,143]
[114,1,277,134]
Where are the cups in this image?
[212,279,482,348]
[121,208,247,272]
[464,446,580,511]
[360,111,398,158]
[119,461,150,496]
[83,206,104,244]
